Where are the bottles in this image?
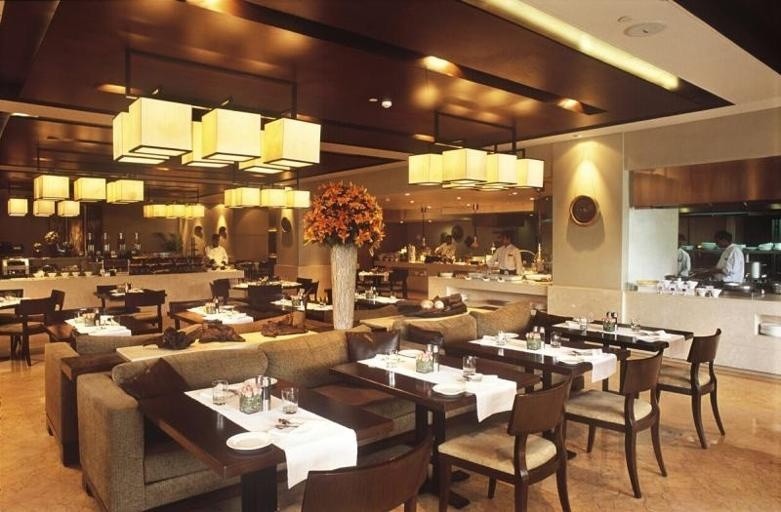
[102,233,110,252]
[86,232,96,255]
[416,343,439,374]
[291,292,303,307]
[203,295,222,315]
[131,232,142,250]
[372,285,377,298]
[240,374,270,414]
[127,277,132,291]
[76,307,112,330]
[117,232,126,250]
[527,326,545,350]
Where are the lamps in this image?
[408,149,546,192]
[143,204,206,220]
[223,187,311,211]
[9,197,82,215]
[112,98,322,175]
[34,175,145,205]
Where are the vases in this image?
[330,241,357,330]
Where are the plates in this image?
[224,431,272,452]
[469,273,542,281]
[243,377,277,388]
[432,382,466,397]
[399,349,422,357]
[502,333,519,338]
[738,241,781,251]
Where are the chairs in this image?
[1,270,412,368]
[437,367,576,512]
[640,327,725,450]
[562,349,664,498]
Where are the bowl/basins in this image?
[83,271,91,277]
[684,245,693,251]
[73,271,81,277]
[33,273,41,278]
[660,279,721,298]
[61,273,69,278]
[440,272,453,278]
[49,272,57,278]
[703,242,717,251]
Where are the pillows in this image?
[105,347,269,384]
[517,306,573,340]
[117,360,204,450]
[408,323,446,353]
[409,313,476,352]
[261,325,367,393]
[345,330,401,386]
[468,302,528,338]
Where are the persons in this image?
[710,229,745,285]
[433,234,456,260]
[479,232,523,276]
[677,235,691,277]
[205,233,230,305]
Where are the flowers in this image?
[301,181,384,256]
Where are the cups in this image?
[279,291,286,303]
[365,290,371,299]
[495,329,507,346]
[384,348,398,366]
[565,312,642,337]
[211,380,229,407]
[461,355,480,376]
[549,331,561,349]
[281,386,299,415]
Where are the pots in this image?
[746,260,762,279]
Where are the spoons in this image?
[276,424,298,429]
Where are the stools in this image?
[129,263,202,273]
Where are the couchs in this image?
[45,327,367,467]
[77,323,438,512]
[301,430,431,512]
[394,301,590,421]
